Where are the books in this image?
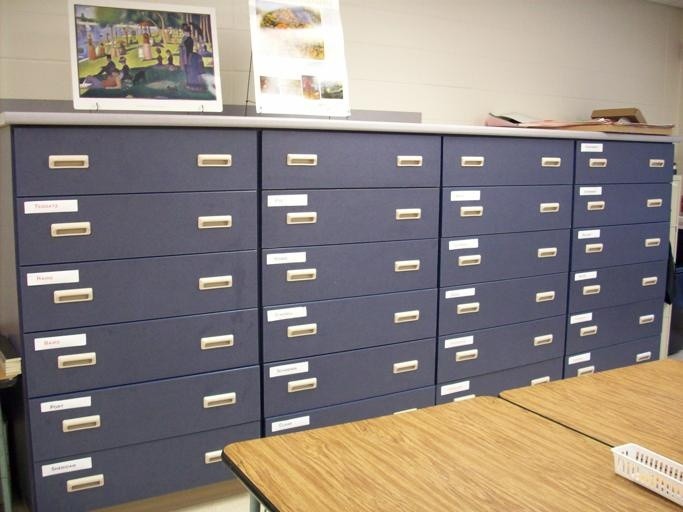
[545,122,673,137]
[590,108,646,123]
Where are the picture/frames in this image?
[247,1,352,119]
[65,0,226,115]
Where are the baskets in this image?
[610,442,683,506]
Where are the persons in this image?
[79,27,213,92]
